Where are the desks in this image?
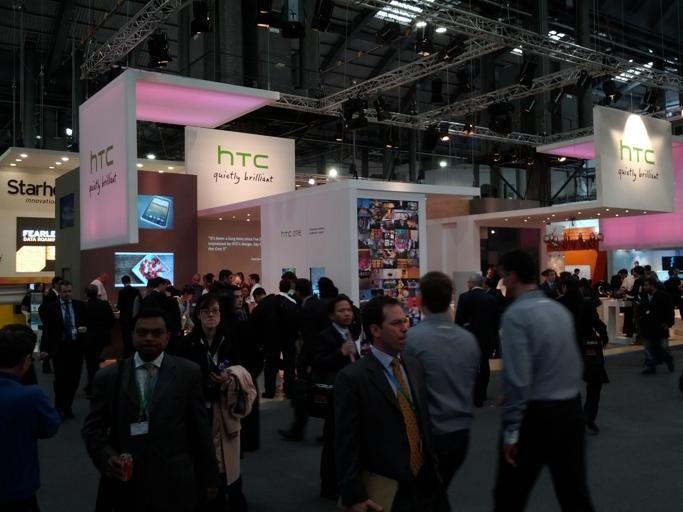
[598,296,632,345]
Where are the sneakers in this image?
[278,423,305,440]
[585,421,598,433]
[262,392,275,399]
[642,367,655,374]
[666,355,674,372]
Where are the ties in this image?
[142,362,158,400]
[346,330,356,361]
[392,359,422,477]
[63,303,75,332]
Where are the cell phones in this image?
[140,195,171,228]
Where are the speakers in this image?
[311,0,335,33]
[281,22,307,39]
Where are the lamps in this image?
[373,97,392,121]
[149,32,170,63]
[462,114,473,135]
[255,0,336,39]
[439,120,450,144]
[414,14,428,29]
[335,123,343,142]
[432,19,447,36]
[415,28,431,56]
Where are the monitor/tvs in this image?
[661,255,683,270]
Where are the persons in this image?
[0,322,62,512]
[493,247,594,512]
[454,261,682,437]
[356,199,422,355]
[330,293,440,512]
[81,308,203,512]
[399,270,482,510]
[20,261,367,464]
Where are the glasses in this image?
[202,308,219,315]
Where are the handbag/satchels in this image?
[598,356,617,382]
